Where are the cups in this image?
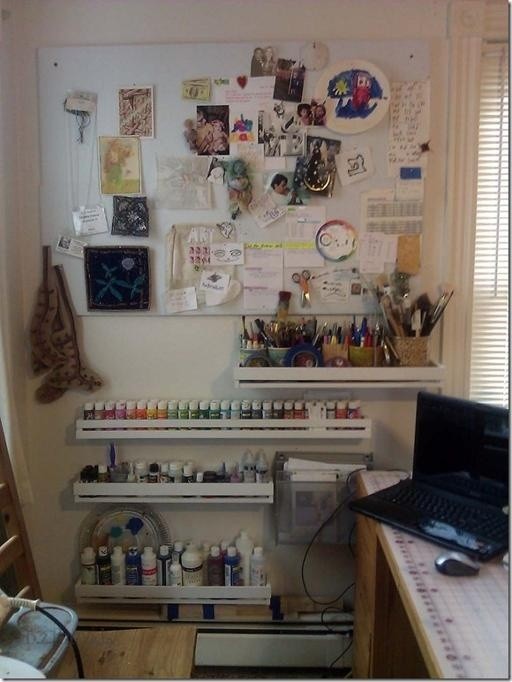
[240,346,271,368]
[391,335,431,367]
[324,343,348,359]
[350,343,380,366]
[267,346,289,366]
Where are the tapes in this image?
[292,269,313,307]
[242,354,274,367]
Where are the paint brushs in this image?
[239,315,328,348]
[376,282,455,337]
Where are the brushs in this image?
[277,291,292,322]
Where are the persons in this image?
[297,101,326,126]
[183,119,197,150]
[274,60,299,102]
[264,128,274,156]
[208,119,227,155]
[262,46,276,76]
[197,109,226,156]
[206,159,304,205]
[251,48,265,76]
[307,138,336,185]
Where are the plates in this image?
[242,353,276,367]
[81,504,172,550]
[324,355,352,367]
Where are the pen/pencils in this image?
[323,317,401,365]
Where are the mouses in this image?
[434,551,480,577]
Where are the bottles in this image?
[78,531,266,586]
[275,291,292,317]
[80,448,269,485]
[83,399,360,433]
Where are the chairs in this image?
[0,422,197,679]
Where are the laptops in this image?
[347,392,508,562]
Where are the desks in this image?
[352,470,508,678]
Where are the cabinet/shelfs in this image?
[73,358,445,607]
